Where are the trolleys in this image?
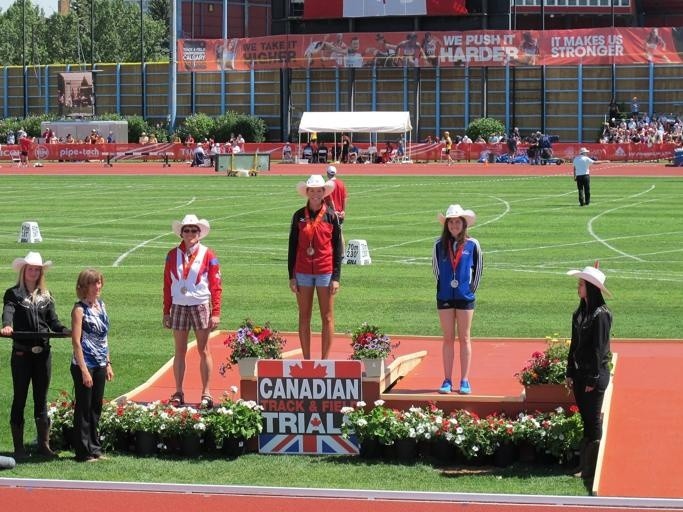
[228,147,260,176]
[534,146,564,165]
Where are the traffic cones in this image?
[482,158,489,167]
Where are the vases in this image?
[359,358,387,379]
[523,382,572,402]
[233,354,264,382]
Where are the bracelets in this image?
[106,360,113,365]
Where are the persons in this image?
[302,33,540,68]
[139,131,245,168]
[69,269,115,462]
[572,147,610,206]
[432,203,482,396]
[283,141,292,160]
[288,174,342,361]
[425,131,455,166]
[565,266,613,478]
[161,215,222,410]
[324,165,346,260]
[303,134,403,163]
[644,28,672,63]
[598,113,683,163]
[0,252,70,460]
[8,127,113,167]
[457,127,551,165]
[216,42,235,70]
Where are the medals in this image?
[306,247,315,256]
[450,279,459,288]
[180,287,187,294]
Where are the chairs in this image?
[279,142,416,166]
[9,148,22,167]
[190,152,207,169]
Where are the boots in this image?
[8,419,30,461]
[35,418,59,459]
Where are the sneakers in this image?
[580,200,591,206]
[199,395,214,410]
[439,379,453,394]
[79,453,111,465]
[168,392,185,407]
[459,379,471,394]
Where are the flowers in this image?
[216,317,289,377]
[46,383,266,454]
[347,321,405,368]
[512,331,617,398]
[333,395,587,463]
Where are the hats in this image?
[578,147,589,154]
[14,251,50,271]
[296,175,335,197]
[326,166,338,175]
[173,214,210,240]
[438,205,477,225]
[566,265,614,299]
